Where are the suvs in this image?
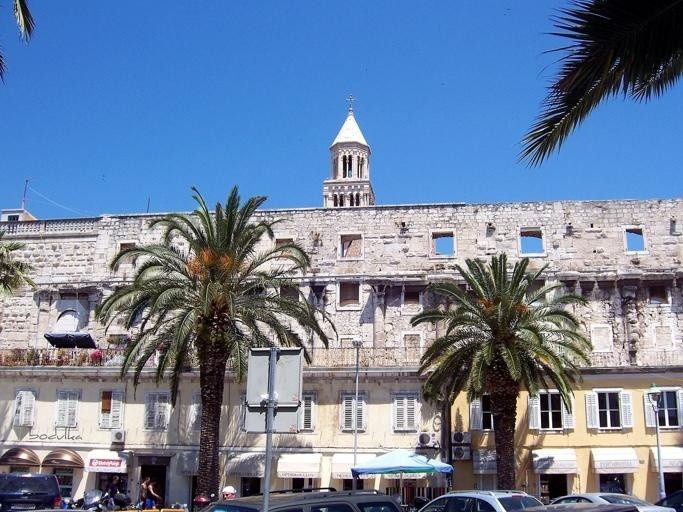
[204,489,403,511]
[415,489,546,510]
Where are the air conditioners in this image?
[414,431,471,461]
[110,429,124,443]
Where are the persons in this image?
[221,485,237,500]
[133,476,151,508]
[143,477,162,509]
[105,475,120,507]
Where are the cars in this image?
[552,492,676,511]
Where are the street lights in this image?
[647,382,667,504]
[350,338,365,487]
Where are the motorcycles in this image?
[59,487,188,511]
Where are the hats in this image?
[222,486,237,495]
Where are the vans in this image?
[0,471,61,511]
